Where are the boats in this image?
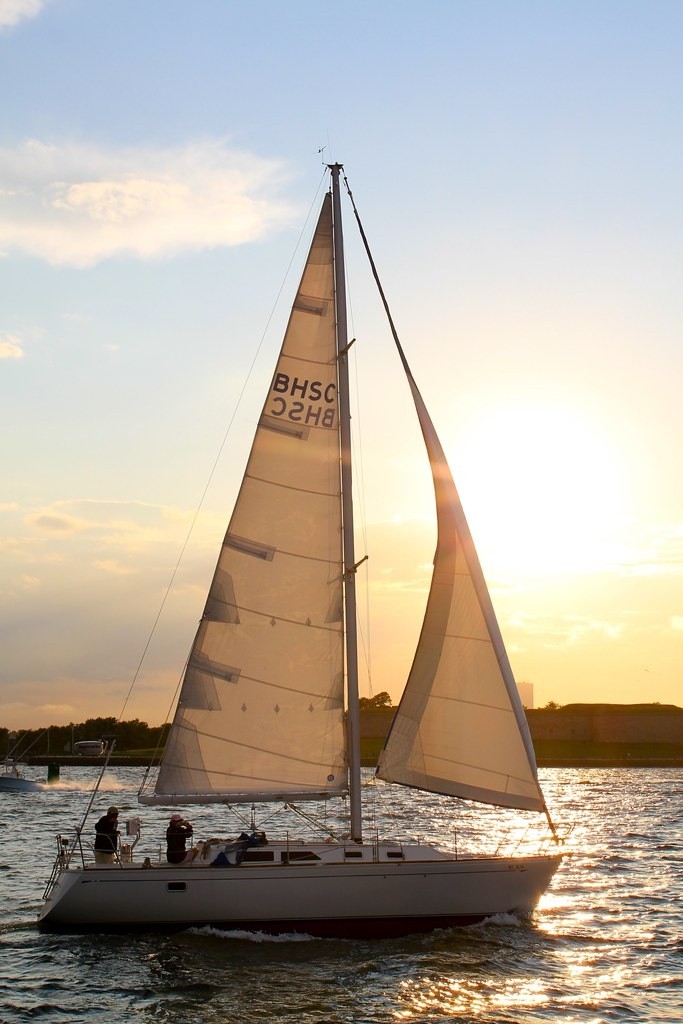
[1,760,48,793]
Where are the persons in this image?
[94,806,121,863]
[166,815,199,864]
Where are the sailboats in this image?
[37,158,572,938]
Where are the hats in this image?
[172,813,182,822]
[107,806,120,814]
